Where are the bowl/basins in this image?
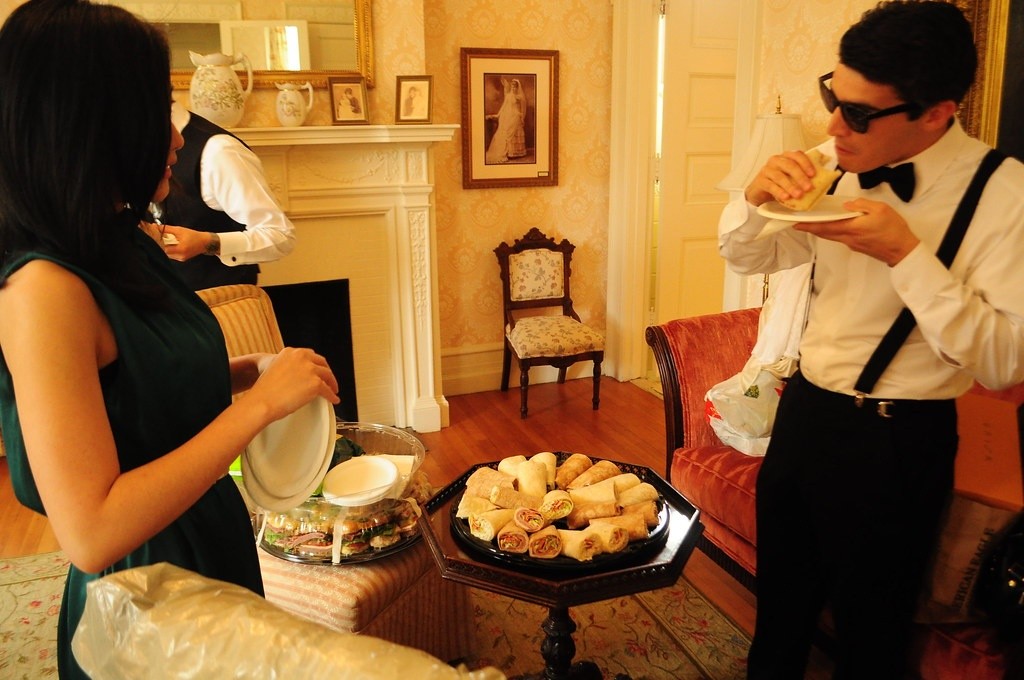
[278,422,426,521]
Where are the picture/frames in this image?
[217,20,311,71]
[460,47,559,189]
[395,75,432,124]
[327,77,370,125]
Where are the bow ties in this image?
[857,162,914,202]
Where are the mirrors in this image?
[170,2,379,91]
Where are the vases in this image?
[187,51,253,130]
[274,81,313,127]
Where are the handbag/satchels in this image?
[971,514,1024,619]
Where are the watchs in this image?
[203,232,220,256]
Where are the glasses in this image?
[818,72,921,132]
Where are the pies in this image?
[778,150,841,212]
[456,452,661,562]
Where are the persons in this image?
[339,88,360,120]
[140,98,294,293]
[715,0,1024,680]
[406,87,416,114]
[0,0,339,680]
[486,79,526,163]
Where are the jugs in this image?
[275,82,314,126]
[189,51,253,128]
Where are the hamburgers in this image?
[261,476,433,557]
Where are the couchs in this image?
[646,306,1024,680]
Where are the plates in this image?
[451,489,671,571]
[757,195,863,222]
[254,499,424,565]
[241,400,336,511]
[323,456,399,507]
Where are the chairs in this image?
[196,283,477,660]
[495,227,604,418]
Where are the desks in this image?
[420,452,705,679]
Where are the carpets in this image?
[1,550,760,680]
